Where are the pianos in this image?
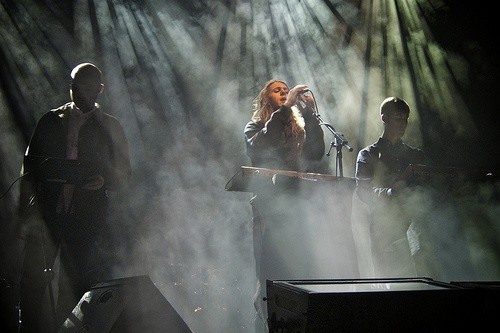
[225,165,357,192]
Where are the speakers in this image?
[57,275,191,333]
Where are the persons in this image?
[20,63,131,327]
[355,97,435,281]
[242,80,325,332]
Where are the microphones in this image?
[296,89,309,96]
